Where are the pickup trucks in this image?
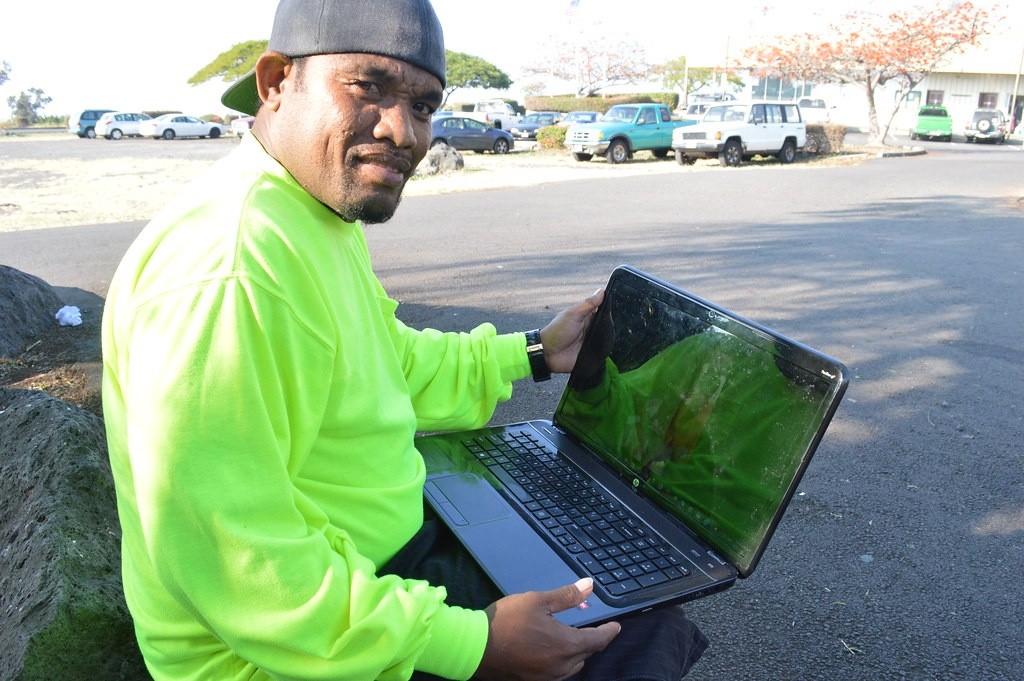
[563,103,699,164]
[909,105,952,141]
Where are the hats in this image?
[221,0,446,117]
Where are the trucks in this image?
[452,99,523,132]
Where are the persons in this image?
[97,0,706,679]
[569,294,833,558]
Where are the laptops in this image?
[414,264,850,630]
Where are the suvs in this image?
[68,109,119,139]
[671,99,807,167]
[966,108,1006,145]
[793,96,830,124]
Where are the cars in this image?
[138,113,225,140]
[509,112,564,141]
[683,103,727,121]
[231,116,255,139]
[551,111,603,127]
[94,112,154,140]
[427,117,514,155]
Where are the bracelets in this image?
[523,328,552,384]
